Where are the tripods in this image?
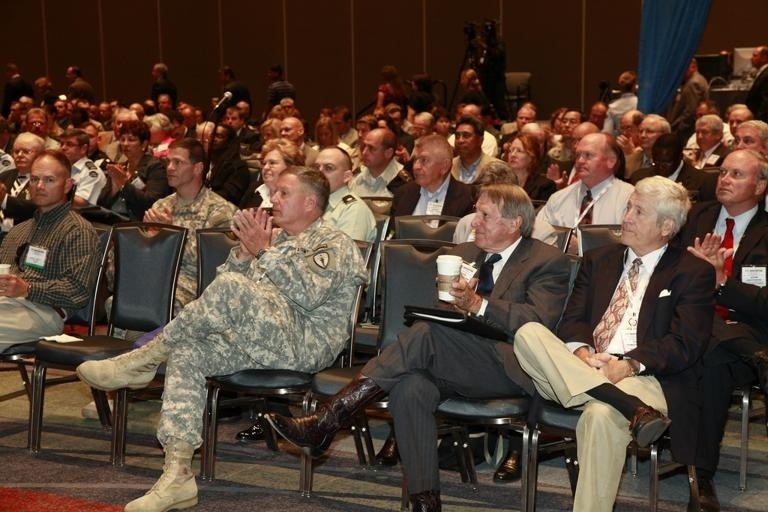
[449,41,514,119]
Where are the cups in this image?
[0,264,10,293]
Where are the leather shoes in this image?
[494,439,541,483]
[234,414,286,442]
[372,430,399,466]
[629,405,670,448]
[689,479,720,512]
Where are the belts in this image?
[53,304,65,318]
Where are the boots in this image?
[266,372,387,460]
[409,490,442,512]
[76,333,172,392]
[124,439,198,512]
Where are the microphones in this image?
[213,90,232,111]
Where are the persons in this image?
[671,58,709,135]
[1,63,768,484]
[669,149,768,512]
[745,45,768,123]
[468,20,508,121]
[513,173,717,512]
[264,183,570,512]
[75,165,369,512]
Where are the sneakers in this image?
[82,401,132,419]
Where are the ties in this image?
[593,258,643,352]
[1,178,21,221]
[477,254,502,296]
[577,190,593,226]
[719,218,734,278]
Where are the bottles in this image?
[435,254,463,303]
